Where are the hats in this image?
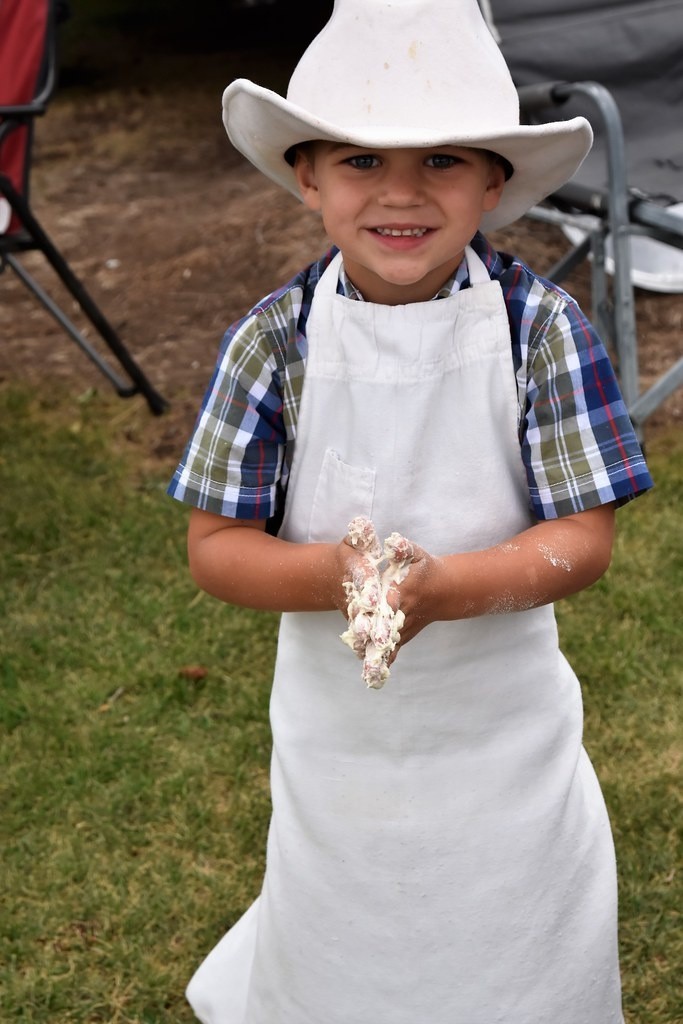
[221,0,598,237]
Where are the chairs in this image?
[478,0,683,466]
[0,41,170,418]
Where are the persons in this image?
[166,0,656,1024]
